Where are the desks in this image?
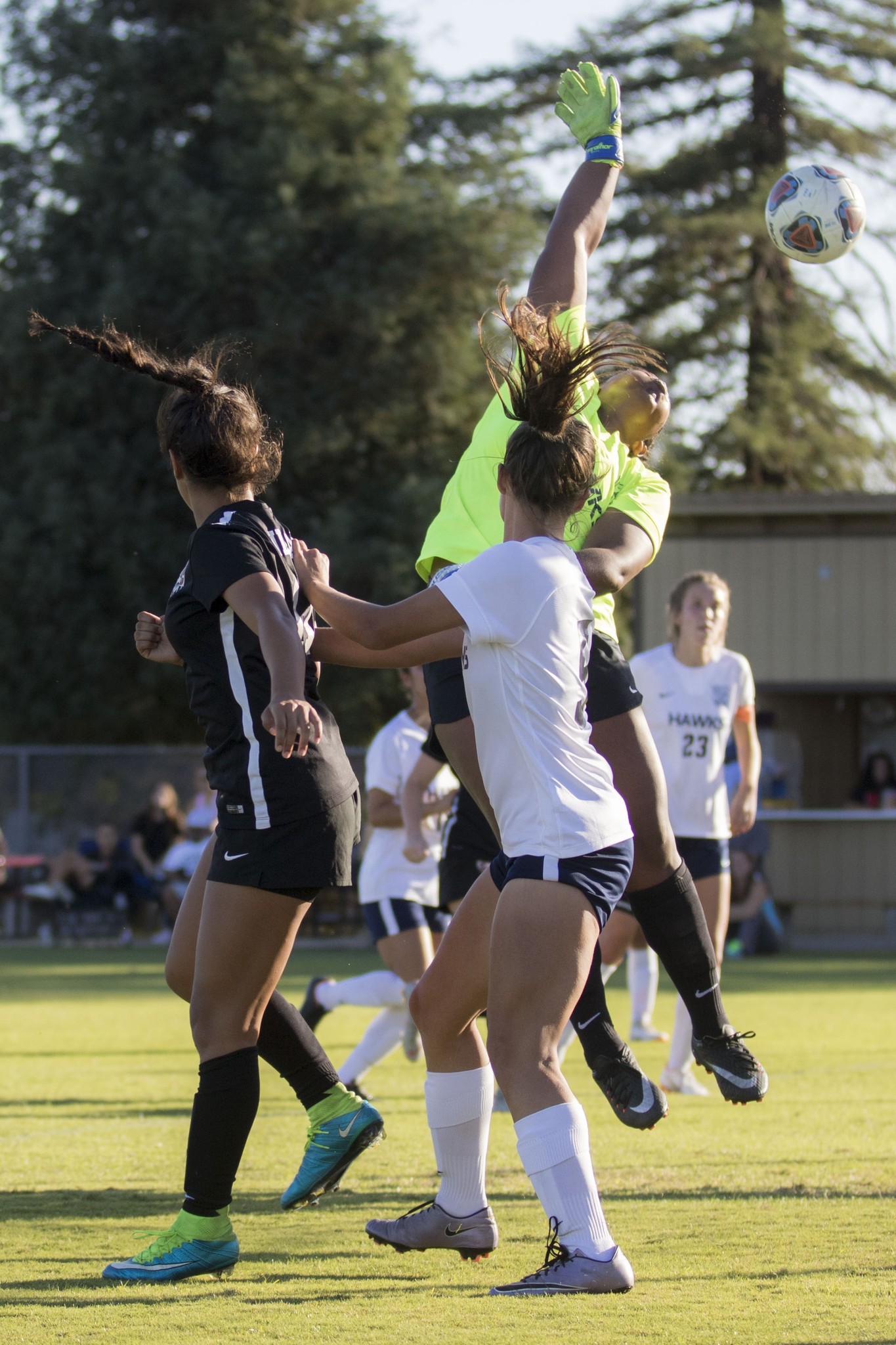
[0,854,49,939]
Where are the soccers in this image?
[766,166,863,264]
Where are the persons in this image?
[0,757,221,952]
[12,298,382,1278]
[722,730,789,857]
[728,849,766,960]
[299,661,461,1101]
[411,61,765,1128]
[850,752,896,811]
[291,276,637,1300]
[493,570,763,1113]
[623,928,668,1044]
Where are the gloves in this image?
[555,61,625,170]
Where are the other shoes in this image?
[120,927,171,944]
[346,1079,369,1101]
[300,977,330,1030]
[661,1066,708,1097]
[21,885,56,900]
[630,1027,670,1042]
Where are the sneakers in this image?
[691,1024,768,1105]
[101,1205,240,1282]
[281,1081,385,1210]
[366,1203,499,1261]
[591,1043,669,1131]
[487,1244,634,1296]
